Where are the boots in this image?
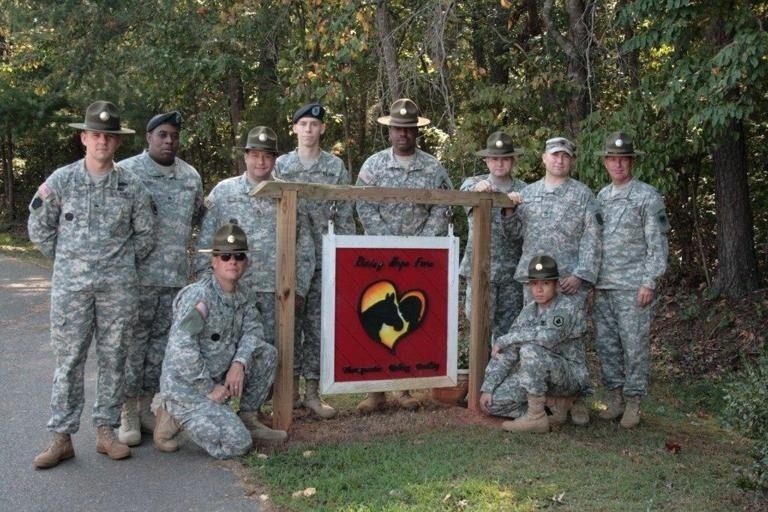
[502,395,589,434]
[292,375,337,418]
[358,392,386,413]
[392,390,419,410]
[600,387,626,420]
[620,395,641,428]
[238,410,288,442]
[35,432,75,468]
[96,393,183,459]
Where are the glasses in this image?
[220,253,245,262]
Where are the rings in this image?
[233,384,238,389]
[225,395,228,401]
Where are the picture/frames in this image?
[319,235,460,396]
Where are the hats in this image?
[292,104,325,124]
[69,100,136,134]
[198,224,263,254]
[594,132,645,157]
[233,126,282,153]
[476,132,523,157]
[147,111,182,132]
[526,255,561,281]
[546,137,577,158]
[377,98,431,128]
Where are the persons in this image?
[500,137,602,425]
[192,125,317,345]
[271,102,357,420]
[28,100,158,468]
[354,98,455,416]
[154,222,288,460]
[593,131,672,428]
[116,109,205,446]
[457,131,528,345]
[479,255,596,434]
